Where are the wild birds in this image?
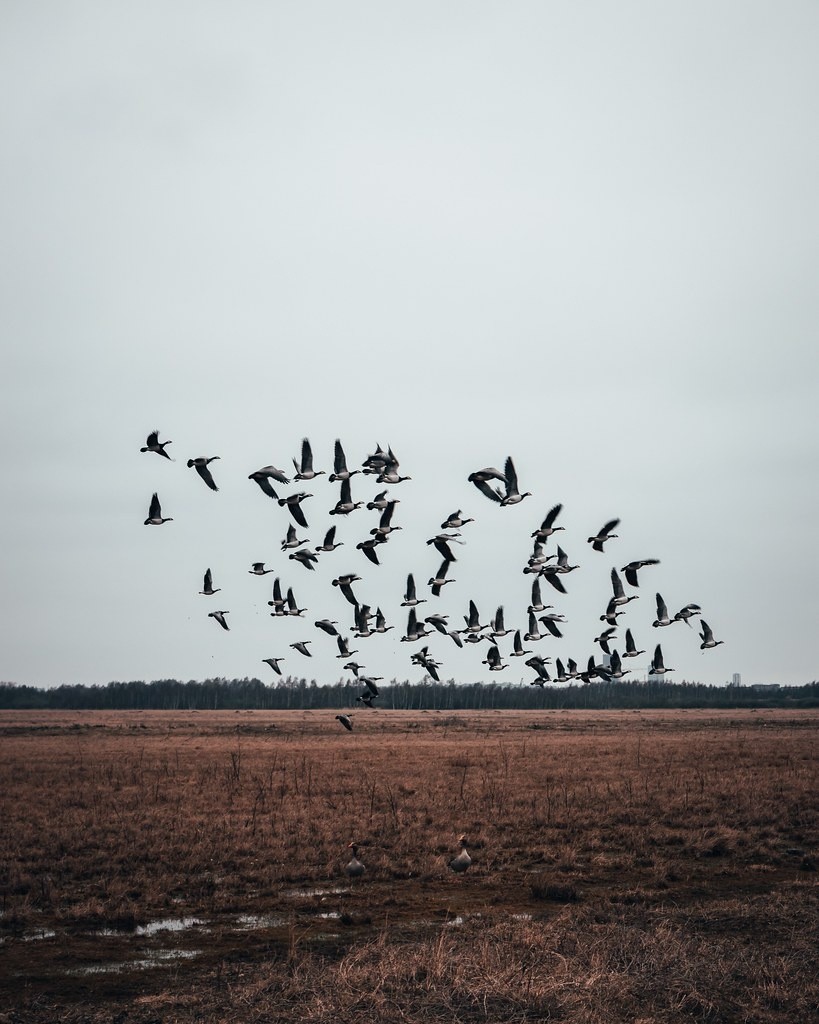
[345,842,366,889]
[199,567,221,597]
[249,438,726,709]
[335,713,355,731]
[139,431,222,526]
[207,610,232,631]
[451,835,472,874]
